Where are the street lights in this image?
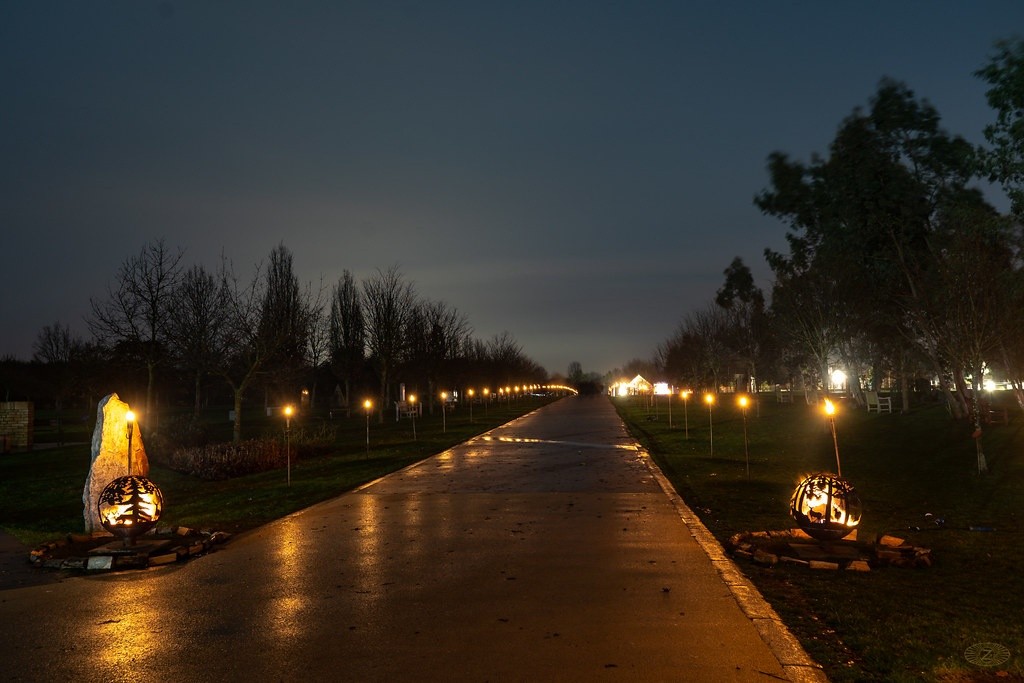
[824,401,843,478]
[466,385,578,427]
[281,404,295,489]
[738,396,751,485]
[409,394,418,442]
[705,394,715,458]
[440,392,447,434]
[125,410,135,477]
[681,391,690,440]
[363,400,372,461]
[607,381,678,429]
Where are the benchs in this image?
[865,391,891,413]
[397,401,419,420]
